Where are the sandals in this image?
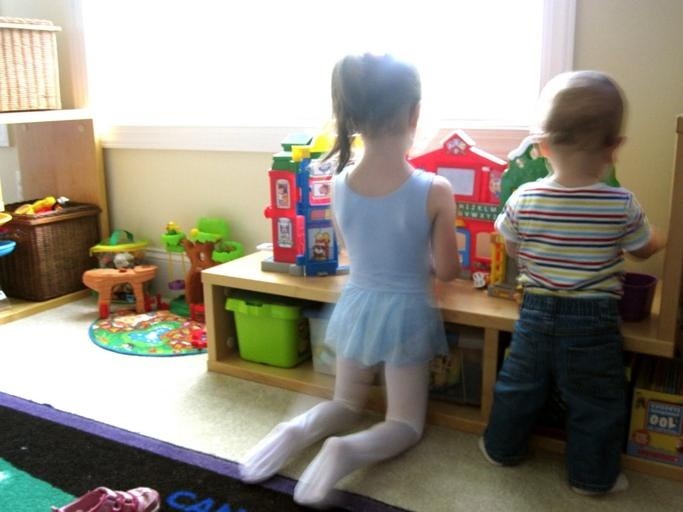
[50,483,162,512]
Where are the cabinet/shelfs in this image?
[0,0,110,330]
[198,111,682,483]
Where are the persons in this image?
[239,53,462,507]
[478,70,667,497]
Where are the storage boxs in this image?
[304,309,374,382]
[625,375,683,467]
[222,295,307,370]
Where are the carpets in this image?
[85,306,208,357]
[1,388,408,512]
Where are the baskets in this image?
[0,193,103,302]
[0,16,67,114]
